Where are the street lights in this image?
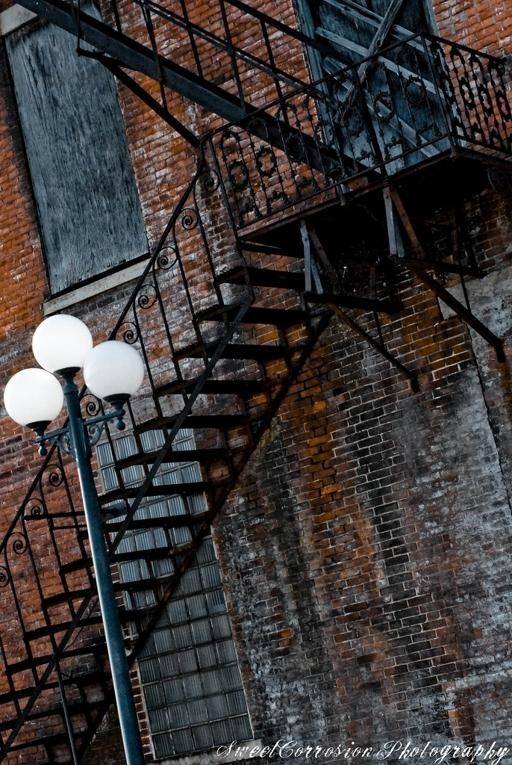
[1,310,153,765]
